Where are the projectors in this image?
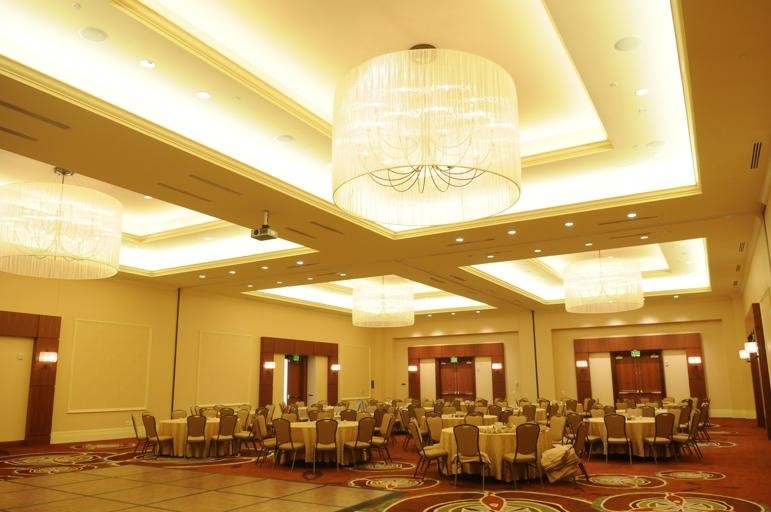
[251,229,277,241]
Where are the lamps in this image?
[349,275,414,328]
[0,162,122,282]
[739,341,759,362]
[38,350,57,368]
[561,249,645,315]
[331,40,521,226]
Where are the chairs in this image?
[132,403,255,459]
[254,398,710,474]
[539,421,590,486]
[408,422,449,479]
[502,423,545,489]
[453,424,493,490]
